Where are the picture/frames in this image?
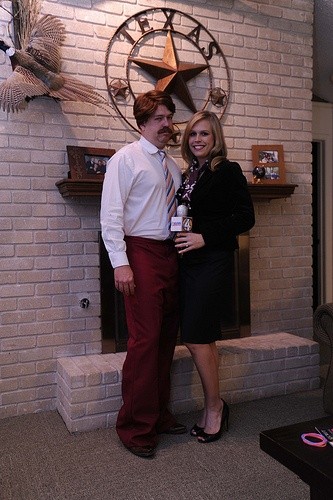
[67,145,116,179]
[252,143,287,186]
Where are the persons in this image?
[100,89,183,460]
[173,111,255,443]
[86,157,107,175]
[260,152,275,163]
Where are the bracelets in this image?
[301,432,328,448]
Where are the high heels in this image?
[189,424,206,437]
[198,397,230,443]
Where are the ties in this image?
[157,151,178,243]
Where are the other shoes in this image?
[127,445,152,456]
[156,420,186,434]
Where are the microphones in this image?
[171,204,192,256]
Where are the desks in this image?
[260,414,332,500]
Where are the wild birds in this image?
[0,14,119,122]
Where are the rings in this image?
[185,242,188,247]
